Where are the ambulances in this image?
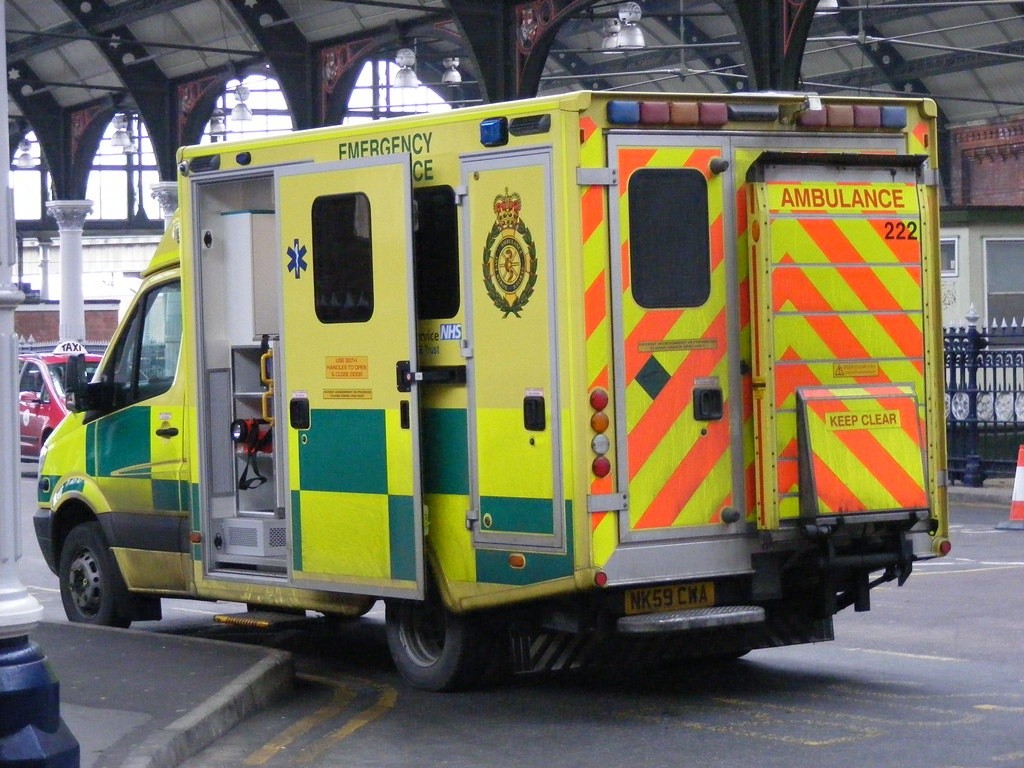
[33,89,952,691]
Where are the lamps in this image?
[231,86,254,121]
[17,138,35,170]
[814,0,840,17]
[123,131,135,155]
[441,57,462,87]
[210,108,226,136]
[393,48,420,88]
[111,116,131,148]
[601,1,646,55]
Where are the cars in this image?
[18,341,148,456]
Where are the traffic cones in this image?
[994,444,1024,528]
